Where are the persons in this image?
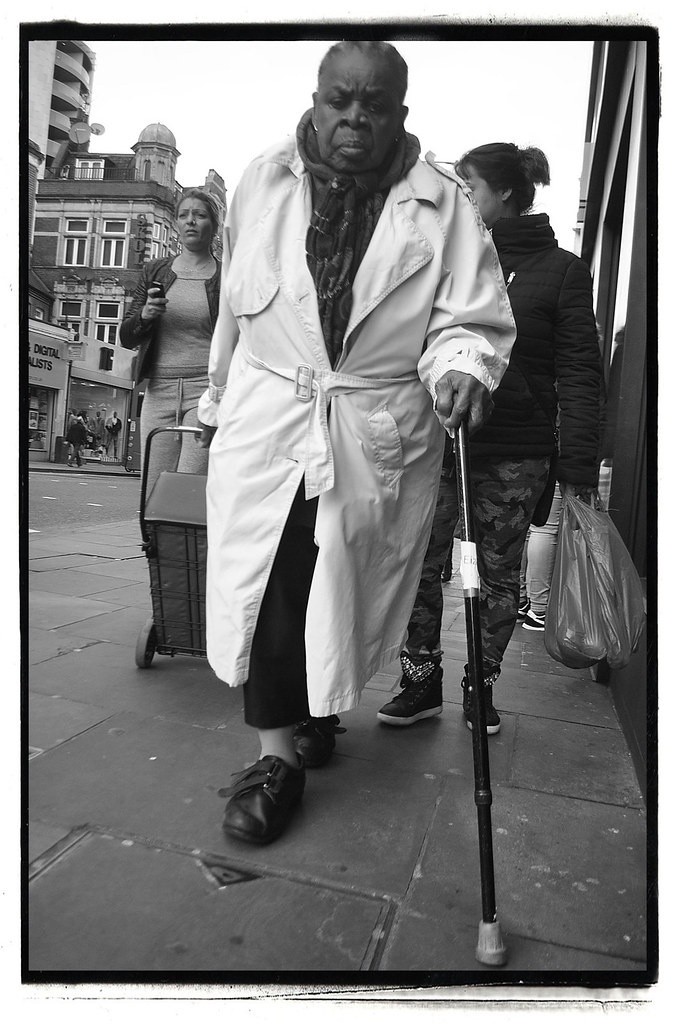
[66,416,87,465]
[195,38,517,848]
[113,188,224,502]
[515,478,563,631]
[375,141,606,735]
[67,408,122,459]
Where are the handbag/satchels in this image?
[530,438,559,527]
[544,491,645,671]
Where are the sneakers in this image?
[377,652,443,726]
[461,662,501,735]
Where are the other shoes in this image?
[294,715,347,769]
[522,609,545,631]
[516,597,531,624]
[67,463,73,467]
[218,752,305,844]
[77,462,82,466]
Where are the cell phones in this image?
[153,281,166,299]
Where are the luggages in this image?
[134,425,216,667]
